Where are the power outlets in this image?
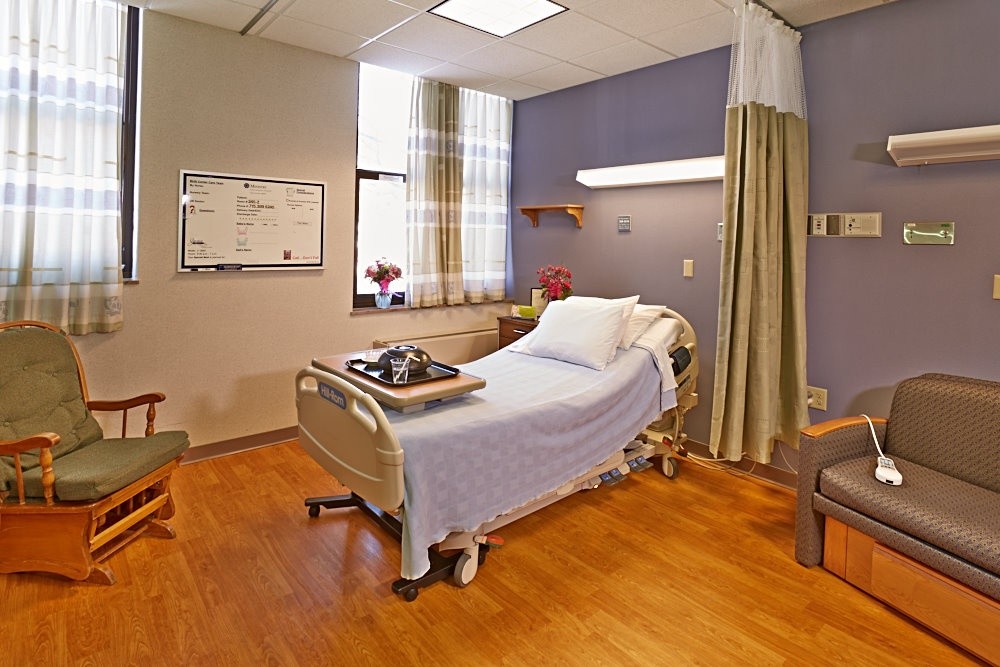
[807,386,828,411]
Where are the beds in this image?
[295,298,702,602]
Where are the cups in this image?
[390,358,411,383]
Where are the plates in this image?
[362,356,380,365]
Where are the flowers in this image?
[365,256,403,294]
[535,265,574,301]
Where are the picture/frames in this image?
[531,289,549,317]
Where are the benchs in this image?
[794,368,1000,667]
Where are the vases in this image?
[375,280,394,308]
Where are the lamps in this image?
[576,155,726,191]
[887,124,1000,167]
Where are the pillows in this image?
[508,295,667,372]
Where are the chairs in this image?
[0,319,191,585]
